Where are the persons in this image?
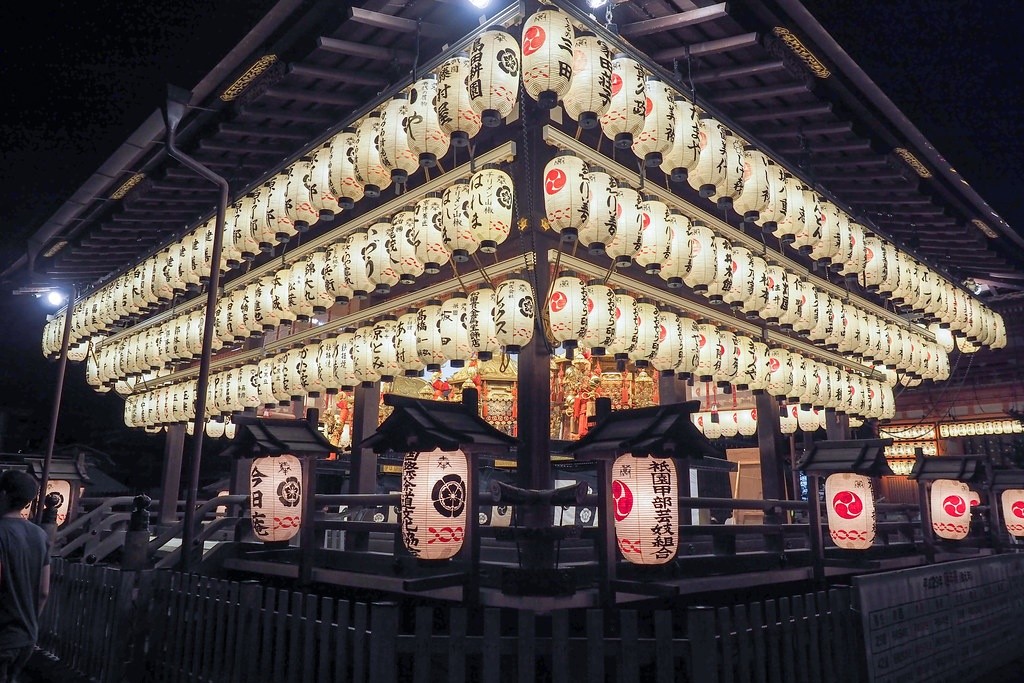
[0,469,50,683]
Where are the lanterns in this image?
[19,480,71,527]
[402,449,467,560]
[825,473,876,549]
[931,480,970,540]
[612,454,678,564]
[41,4,1007,440]
[250,454,302,542]
[969,491,980,521]
[940,420,1024,438]
[879,425,936,475]
[1002,489,1024,536]
